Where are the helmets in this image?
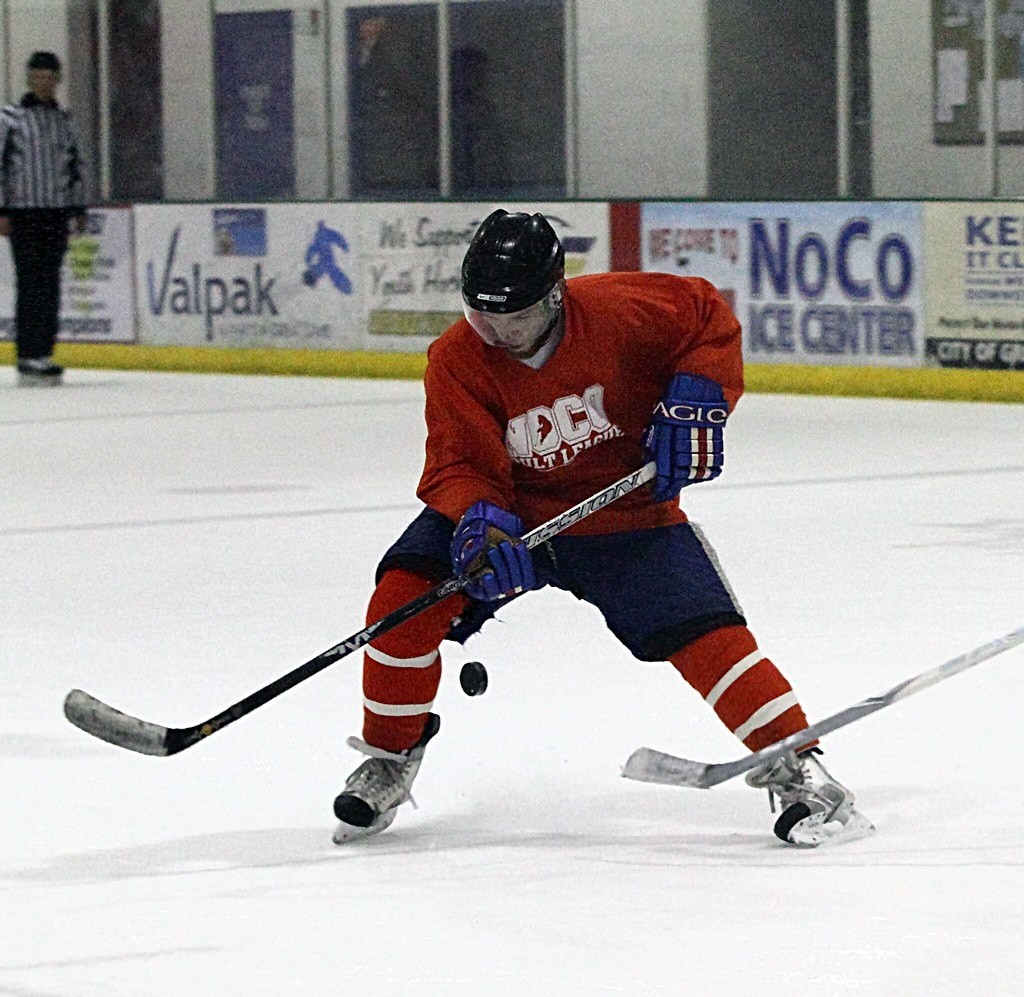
[460,208,566,314]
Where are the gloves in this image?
[641,372,729,505]
[450,499,535,602]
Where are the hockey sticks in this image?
[621,623,1024,789]
[63,462,660,756]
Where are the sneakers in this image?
[745,745,876,848]
[330,712,439,851]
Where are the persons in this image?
[1,48,94,380]
[330,209,852,843]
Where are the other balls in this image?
[458,661,489,696]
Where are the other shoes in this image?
[15,355,68,378]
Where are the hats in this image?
[27,50,61,72]
